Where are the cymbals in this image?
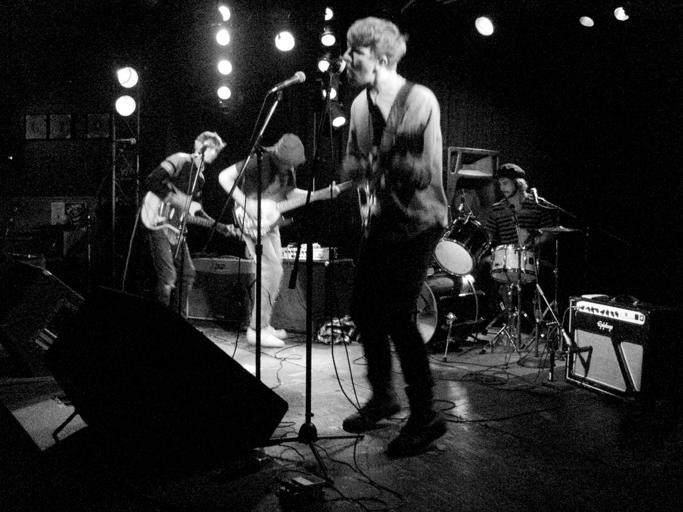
[540,226,580,233]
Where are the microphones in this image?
[268,70,307,96]
[510,204,519,226]
[190,140,210,161]
[329,56,346,75]
[114,134,138,149]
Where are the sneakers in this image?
[342,398,400,433]
[266,326,288,339]
[388,418,446,454]
[246,327,285,348]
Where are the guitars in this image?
[141,191,241,236]
[357,124,424,244]
[234,180,353,241]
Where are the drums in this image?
[433,211,491,276]
[416,272,486,349]
[490,244,539,284]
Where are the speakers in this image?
[566,293,680,406]
[1,258,87,377]
[44,287,292,487]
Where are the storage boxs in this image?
[564,297,678,406]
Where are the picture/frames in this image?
[48,113,73,140]
[24,114,48,141]
[87,113,111,139]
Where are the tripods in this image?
[480,286,525,356]
[528,251,573,358]
[262,117,367,488]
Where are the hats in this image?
[496,163,526,178]
[274,133,306,164]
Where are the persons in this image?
[485,163,560,335]
[217,134,340,348]
[340,16,448,460]
[140,130,236,321]
